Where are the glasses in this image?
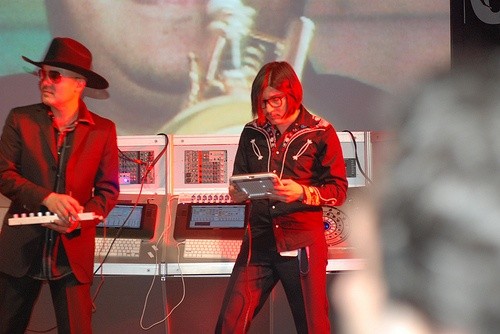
[38,69,85,85]
[260,93,286,108]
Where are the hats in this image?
[21,37,111,91]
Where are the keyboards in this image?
[183,239,242,260]
[93,238,142,257]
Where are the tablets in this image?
[230,173,284,200]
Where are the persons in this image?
[215,61,348,334]
[0,37,119,334]
[0,1,407,334]
